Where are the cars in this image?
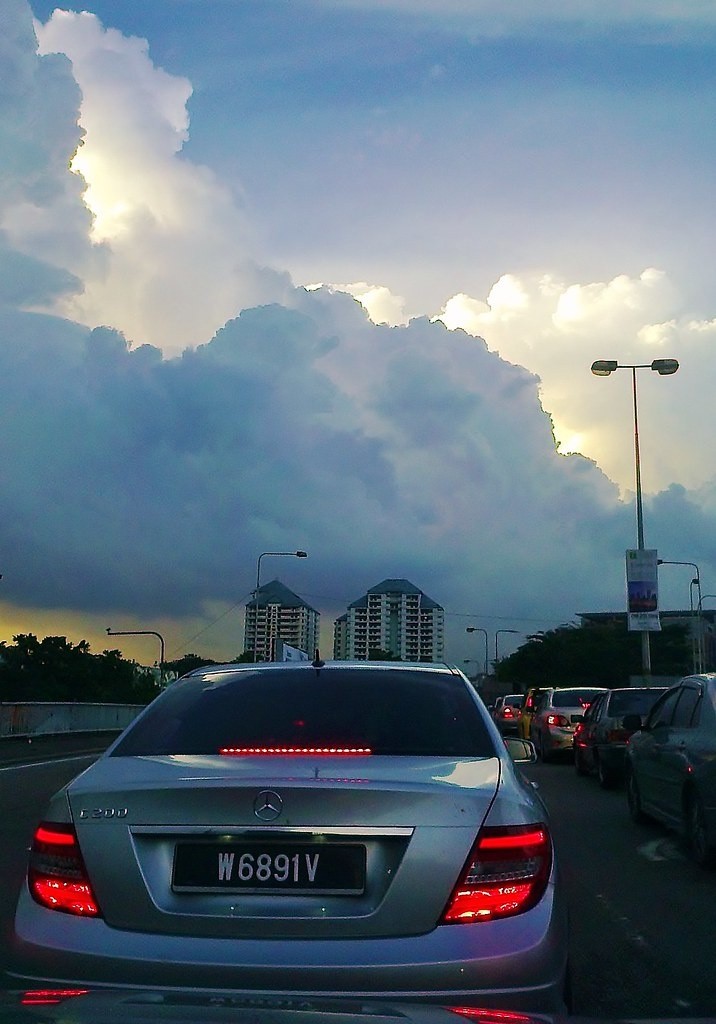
[570,687,671,790]
[487,685,612,765]
[0,659,574,1024]
[622,670,716,873]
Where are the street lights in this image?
[657,560,708,674]
[254,551,307,663]
[495,629,519,664]
[591,358,677,685]
[467,627,488,677]
[690,578,699,674]
[464,659,482,677]
